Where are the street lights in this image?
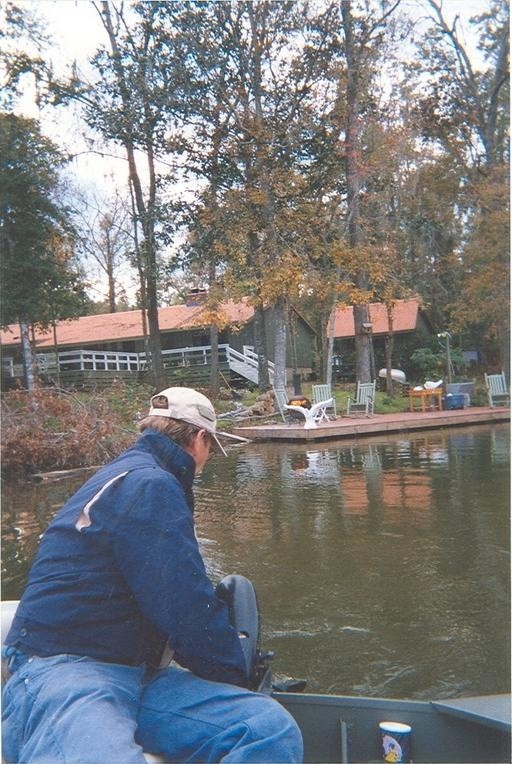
[437,328,452,383]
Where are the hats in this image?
[149,386,230,459]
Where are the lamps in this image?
[361,322,374,332]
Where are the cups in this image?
[378,721,413,763]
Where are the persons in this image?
[1,386,306,764]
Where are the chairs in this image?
[483,370,509,409]
[273,378,376,422]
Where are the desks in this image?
[408,387,443,412]
